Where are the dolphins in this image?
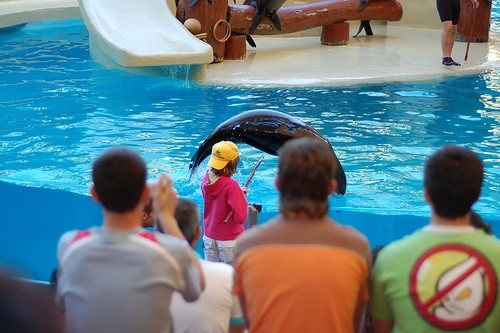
[242,0,289,48]
[188,109,347,196]
[352,0,373,37]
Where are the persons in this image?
[51,149,246,333]
[369,145,500,333]
[201,140,249,264]
[232,138,372,333]
[435,0,479,66]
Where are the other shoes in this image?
[442,57,461,68]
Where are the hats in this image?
[208,141,239,170]
